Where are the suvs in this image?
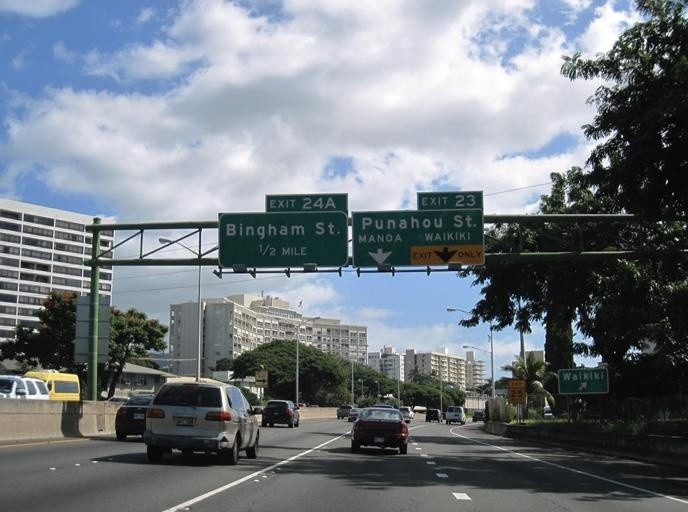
[143,380,265,463]
[426,408,442,422]
[446,406,467,424]
[399,406,415,419]
[473,410,484,422]
[261,399,301,427]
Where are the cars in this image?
[116,394,156,442]
[543,405,552,417]
[337,405,410,454]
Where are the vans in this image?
[23,369,82,403]
[0,374,51,399]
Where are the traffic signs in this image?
[558,369,608,395]
[353,209,482,269]
[265,193,348,213]
[416,191,483,208]
[219,211,347,269]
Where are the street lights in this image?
[294,316,319,408]
[159,236,218,380]
[447,307,496,417]
[375,379,381,399]
[357,377,364,398]
[386,360,412,407]
[350,352,366,405]
[461,346,495,416]
